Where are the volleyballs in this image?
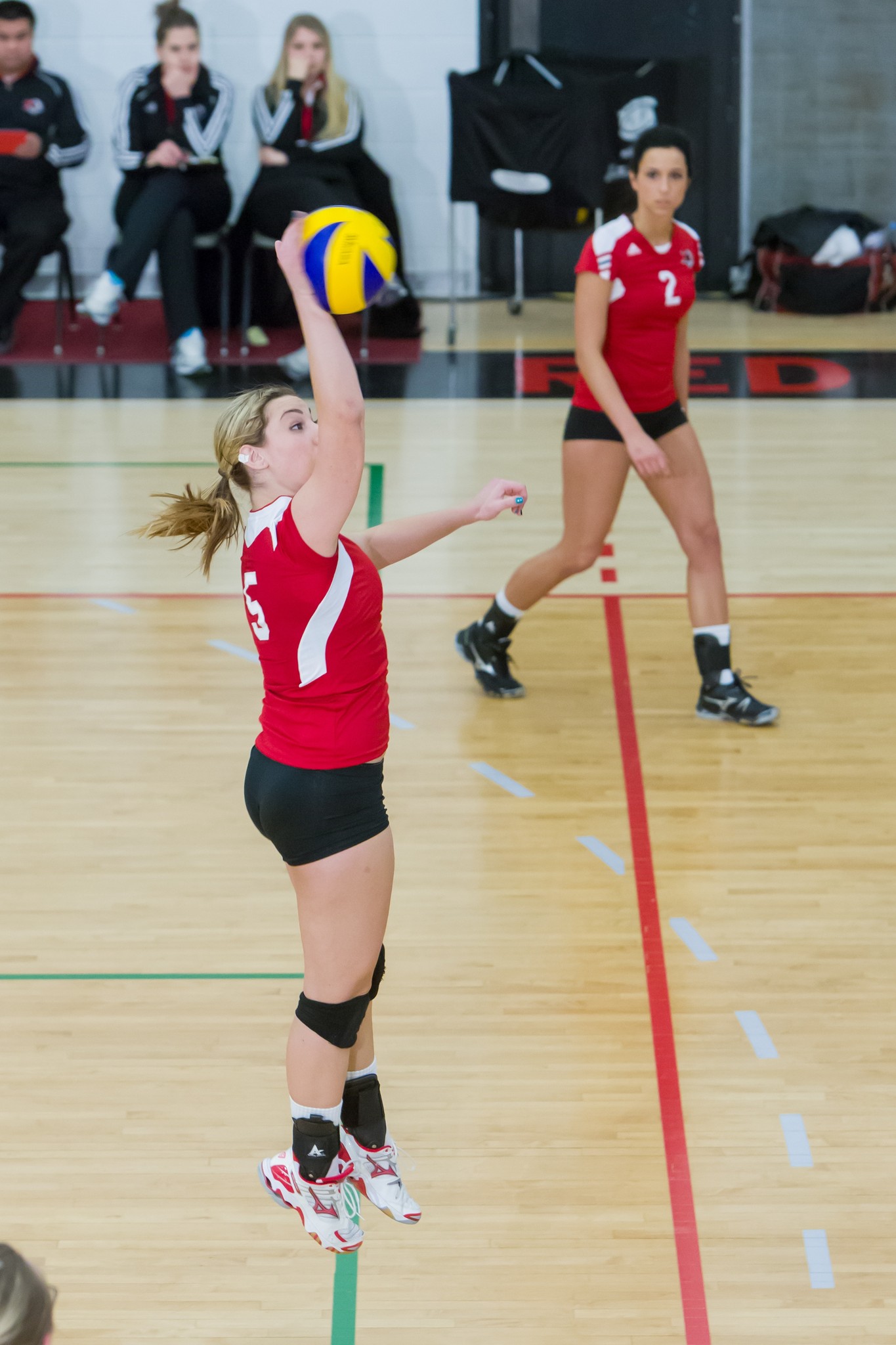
[296,206,397,315]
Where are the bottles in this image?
[862,222,896,251]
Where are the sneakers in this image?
[337,1122,422,1224]
[257,1144,366,1254]
[455,620,527,699]
[693,666,780,726]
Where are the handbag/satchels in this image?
[749,204,896,315]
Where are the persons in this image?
[0,1241,60,1345]
[131,211,528,1256]
[0,0,92,353]
[224,11,420,392]
[72,0,233,376]
[454,124,780,727]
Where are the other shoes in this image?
[74,269,126,326]
[276,345,310,380]
[377,273,410,308]
[169,326,214,377]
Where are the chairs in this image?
[45,222,369,358]
